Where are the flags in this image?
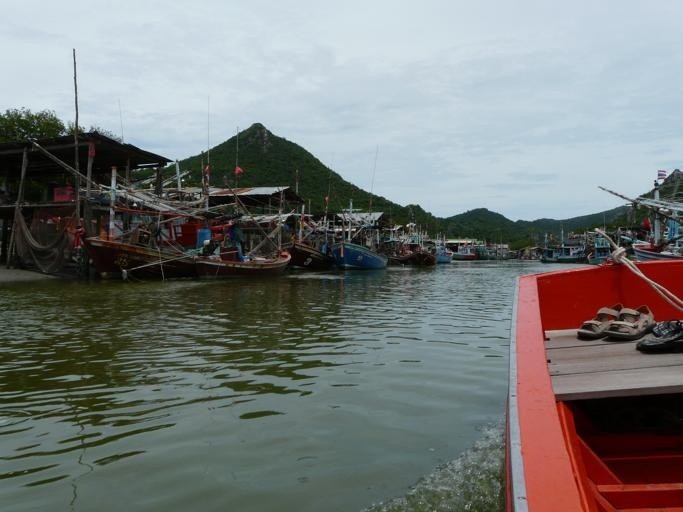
[657,170,666,179]
[234,166,243,173]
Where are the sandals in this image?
[577,304,683,353]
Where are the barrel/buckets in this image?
[197,227,211,247]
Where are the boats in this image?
[501,252,683,512]
